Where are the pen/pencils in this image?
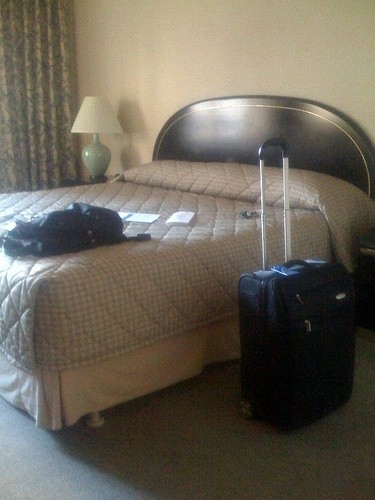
[122,214,133,220]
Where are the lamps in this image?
[70,95,124,184]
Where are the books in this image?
[166,211,195,224]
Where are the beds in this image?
[0,95,374,432]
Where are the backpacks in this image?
[0,203,151,256]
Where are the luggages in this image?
[237,140,355,433]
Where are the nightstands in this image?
[60,180,92,187]
[353,226,375,332]
